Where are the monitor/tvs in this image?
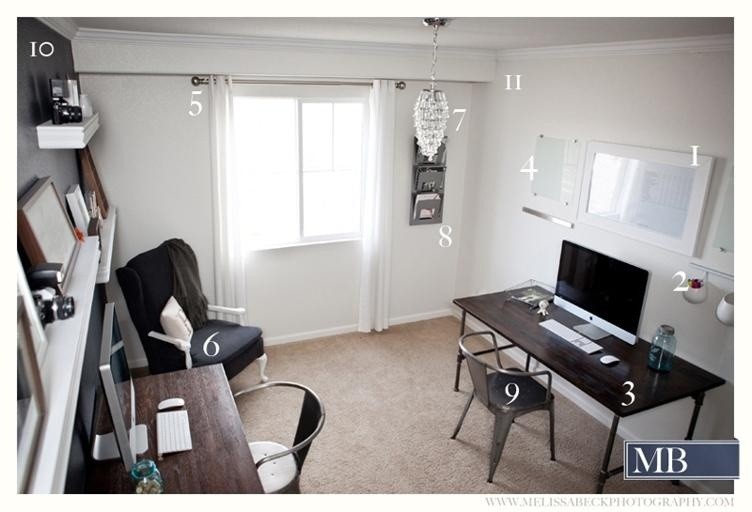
[95,302,148,474]
[555,239,649,346]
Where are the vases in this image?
[716,292,734,327]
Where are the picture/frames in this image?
[576,139,718,259]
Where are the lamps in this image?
[410,19,452,163]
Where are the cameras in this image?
[51,102,83,124]
[32,289,75,319]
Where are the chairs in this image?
[451,331,557,482]
[114,237,269,405]
[232,380,326,493]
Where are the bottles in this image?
[79,93,93,118]
[646,324,677,373]
[130,460,164,494]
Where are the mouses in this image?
[158,397,185,409]
[599,354,618,366]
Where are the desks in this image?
[451,284,727,493]
[92,362,268,494]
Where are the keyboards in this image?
[538,319,602,356]
[156,411,192,454]
